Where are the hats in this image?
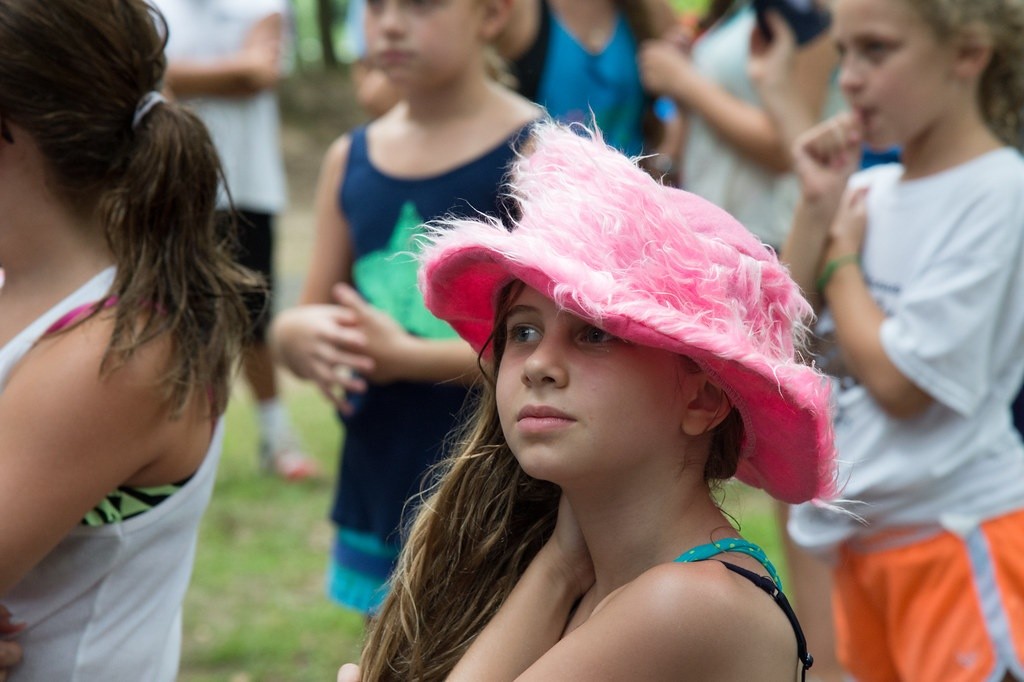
[417,119,843,505]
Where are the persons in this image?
[781,0,1024,682]
[484,0,844,262]
[339,116,846,682]
[0,0,271,682]
[157,0,326,483]
[268,0,555,627]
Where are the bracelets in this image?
[815,256,861,287]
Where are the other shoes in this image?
[262,446,317,480]
